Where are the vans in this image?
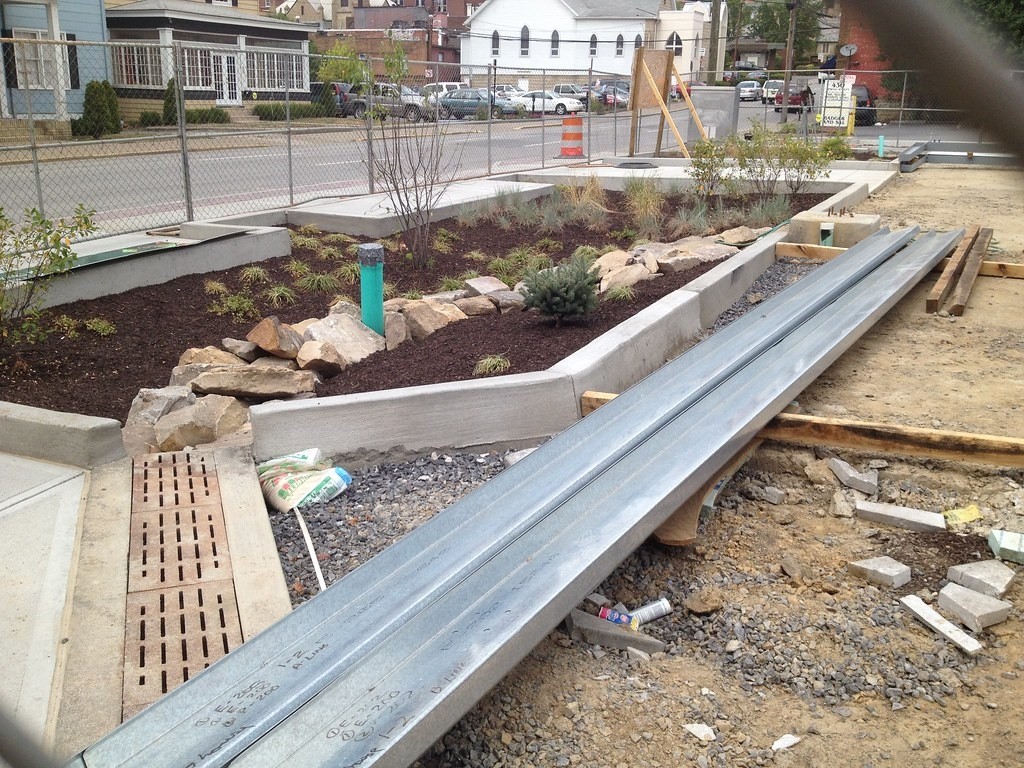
[420,81,471,101]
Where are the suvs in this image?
[309,82,350,119]
[488,83,525,101]
[339,79,444,124]
[761,79,785,104]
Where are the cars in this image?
[429,87,520,121]
[553,78,679,113]
[773,83,816,113]
[744,71,768,80]
[735,80,763,102]
[512,89,584,116]
[722,70,743,82]
[669,79,708,99]
[851,83,880,122]
[729,59,768,70]
